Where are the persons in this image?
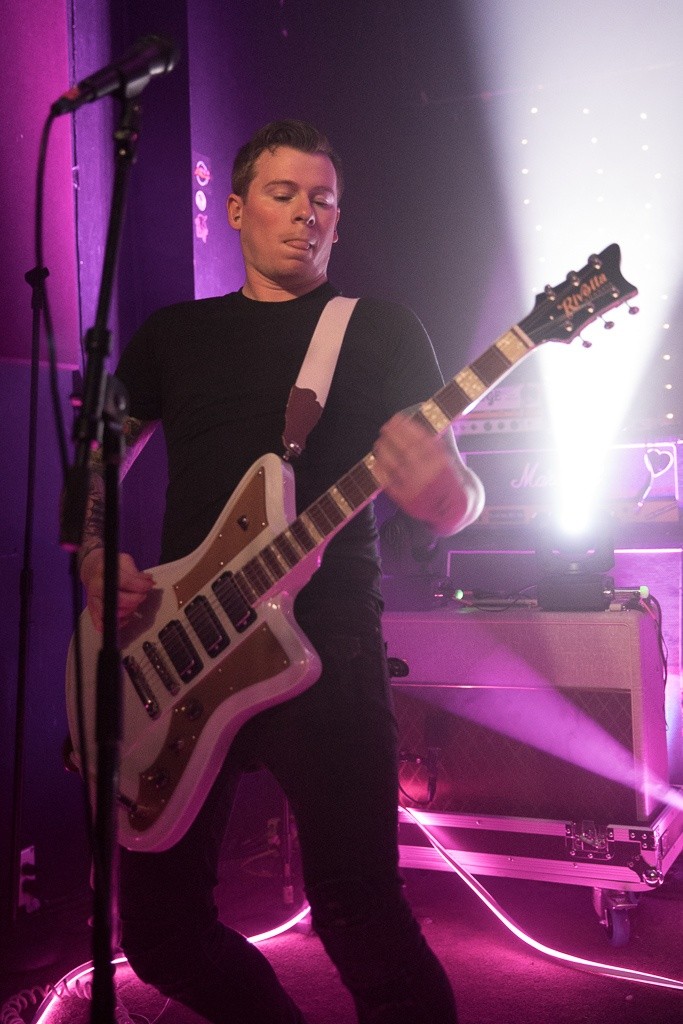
[70,119,489,1024]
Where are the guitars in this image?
[65,245,639,853]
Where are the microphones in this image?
[49,31,181,114]
[424,710,441,795]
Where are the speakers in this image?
[380,617,669,864]
